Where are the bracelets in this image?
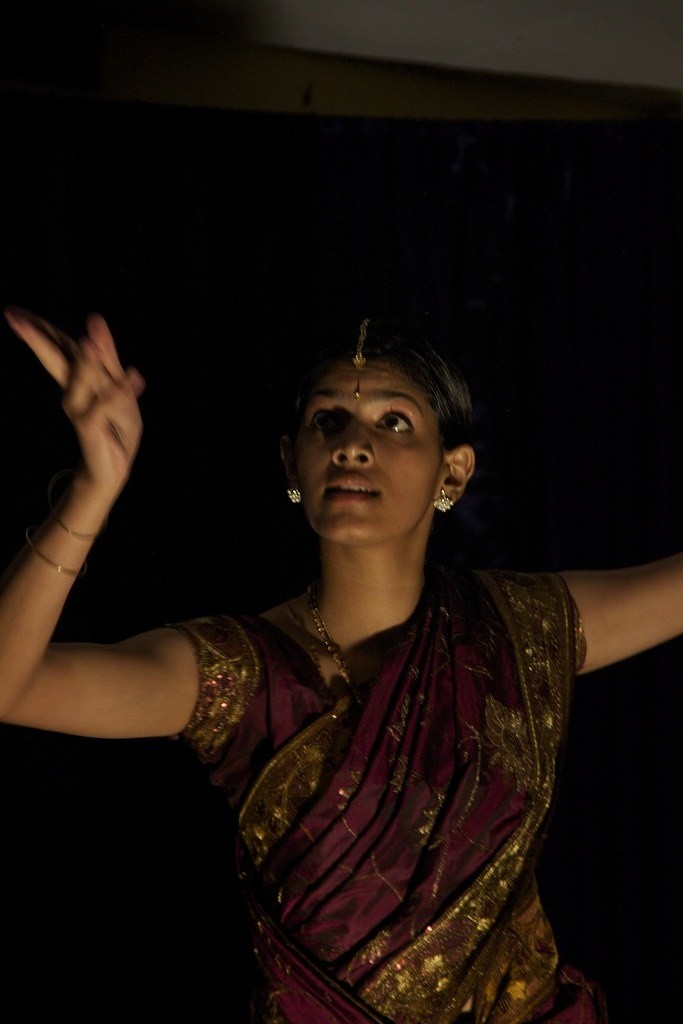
[23,524,89,577]
[45,468,109,546]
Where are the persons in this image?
[0,301,683,1024]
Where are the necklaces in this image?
[282,599,322,678]
[305,577,365,704]
